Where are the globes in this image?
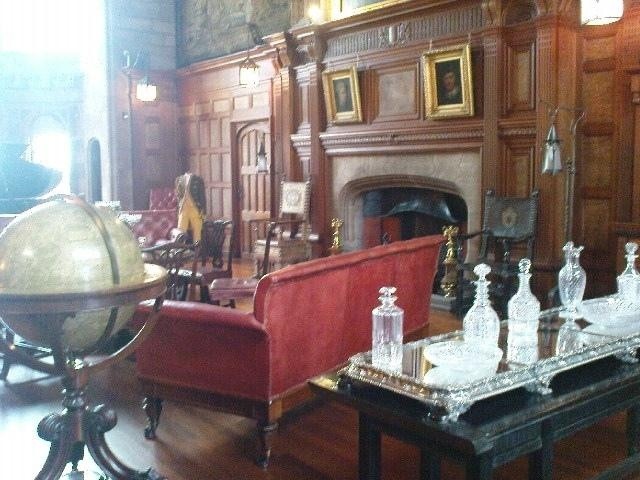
[0,193,170,480]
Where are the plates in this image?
[576,297,639,327]
[424,367,496,388]
[423,340,504,374]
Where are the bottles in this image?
[462,263,501,344]
[371,287,404,360]
[557,240,588,310]
[507,259,541,334]
[615,241,640,302]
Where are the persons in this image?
[442,66,460,100]
[335,79,348,106]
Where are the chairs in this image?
[140,218,234,309]
[249,174,311,278]
[451,190,537,316]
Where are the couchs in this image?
[134,235,444,467]
[122,187,177,247]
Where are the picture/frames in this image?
[321,66,363,123]
[422,43,476,118]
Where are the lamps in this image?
[579,0,626,27]
[240,29,265,87]
[124,49,157,101]
[258,132,270,177]
[541,102,584,243]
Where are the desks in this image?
[308,291,639,478]
